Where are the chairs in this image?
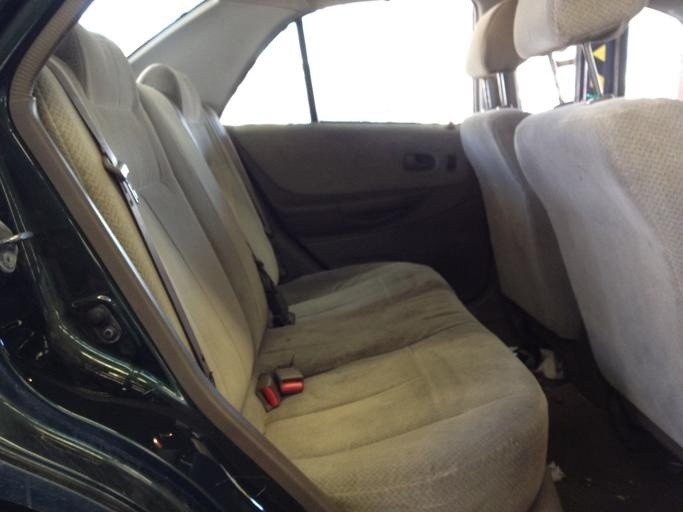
[512,0,683,448]
[458,1,587,344]
[35,21,563,511]
[134,61,470,351]
